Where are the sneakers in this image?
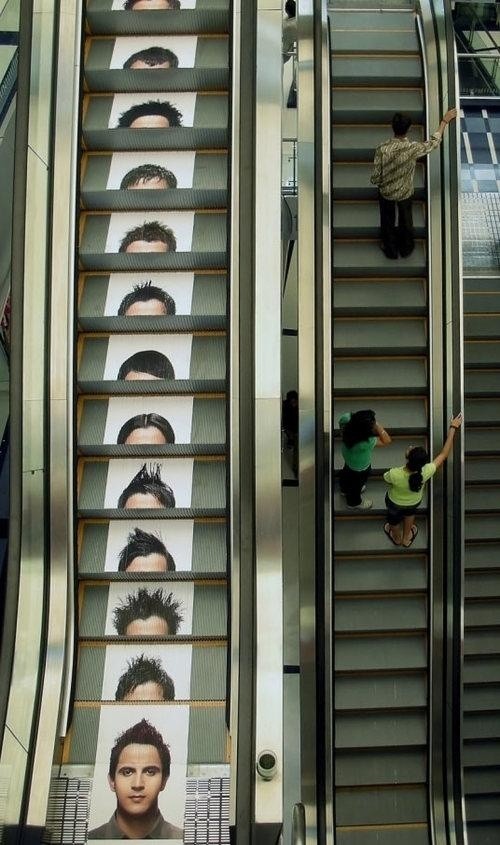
[339,484,368,498]
[344,499,373,511]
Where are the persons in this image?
[370,106,457,260]
[337,409,391,510]
[383,412,463,548]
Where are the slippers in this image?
[383,522,401,545]
[403,523,418,548]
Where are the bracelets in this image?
[379,430,385,437]
[449,425,458,430]
[441,118,448,124]
